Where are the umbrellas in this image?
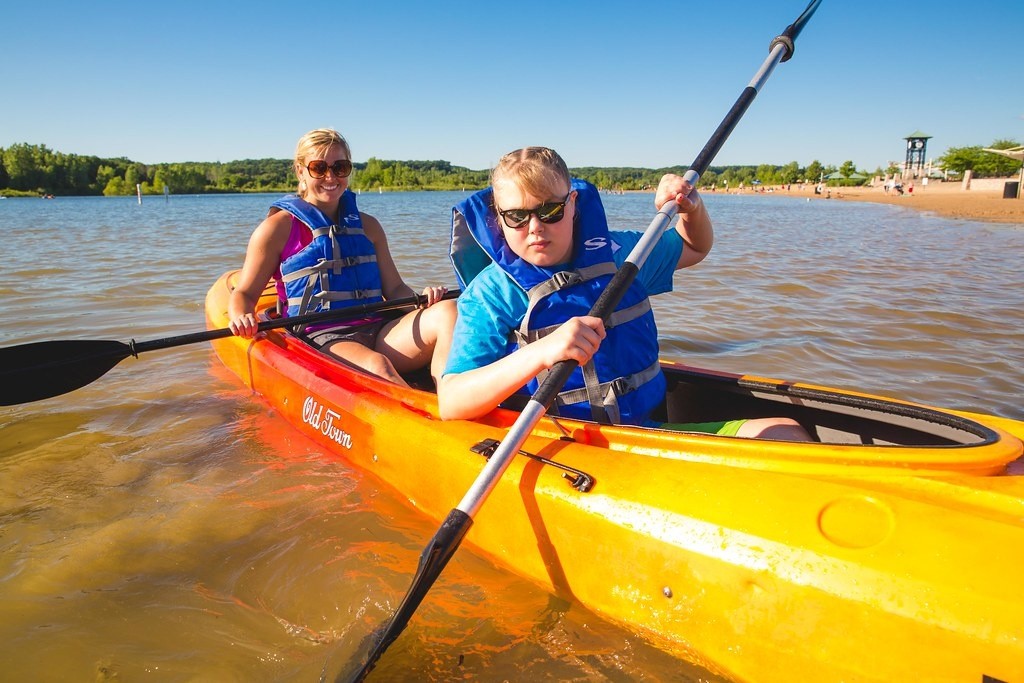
[819,171,868,179]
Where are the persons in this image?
[921,176,929,191]
[226,127,458,392]
[437,147,815,443]
[726,182,806,194]
[883,179,914,196]
[710,182,715,191]
[606,189,624,196]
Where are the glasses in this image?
[302,159,352,179]
[496,191,571,228]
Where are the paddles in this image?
[334,0,824,683]
[0,289,463,407]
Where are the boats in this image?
[200,266,1024,683]
